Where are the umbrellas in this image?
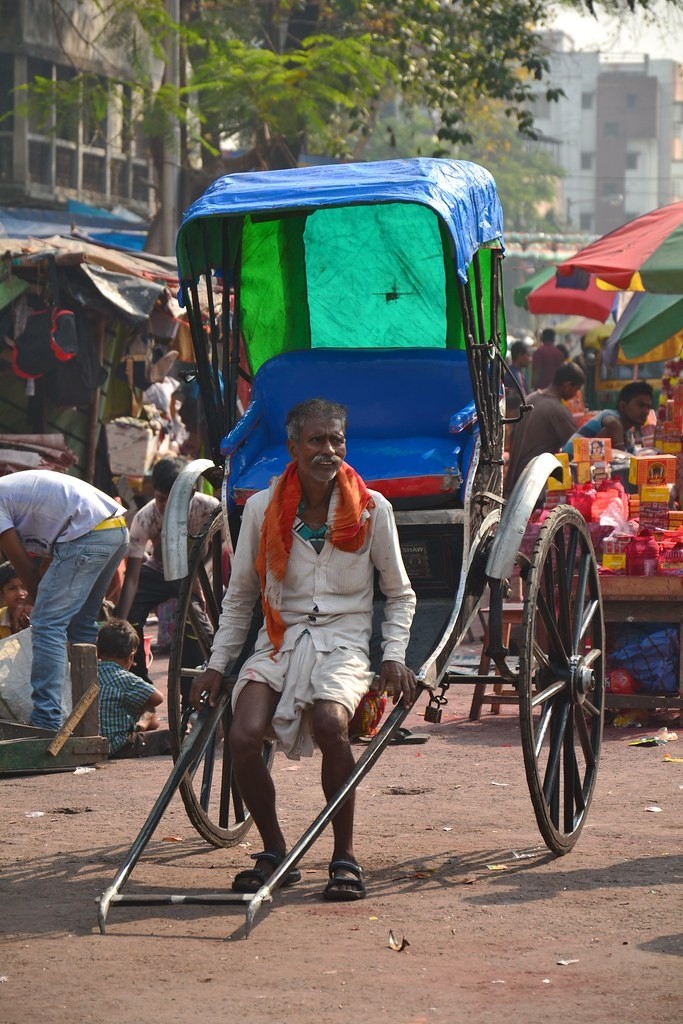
[554,200,683,295]
[601,291,682,374]
[512,264,631,324]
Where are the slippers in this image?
[358,723,430,744]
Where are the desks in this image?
[568,573,683,716]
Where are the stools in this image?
[468,603,552,721]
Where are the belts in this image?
[90,516,128,532]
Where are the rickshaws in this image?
[93,153,607,945]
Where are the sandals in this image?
[231,848,301,892]
[324,857,366,902]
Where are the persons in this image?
[106,456,223,732]
[0,468,131,733]
[188,398,416,901]
[500,328,653,501]
[95,617,172,759]
[0,560,35,641]
[141,361,246,438]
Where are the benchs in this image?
[212,344,501,513]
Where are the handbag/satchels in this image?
[14,255,80,378]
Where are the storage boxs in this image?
[104,423,168,474]
[546,437,683,573]
